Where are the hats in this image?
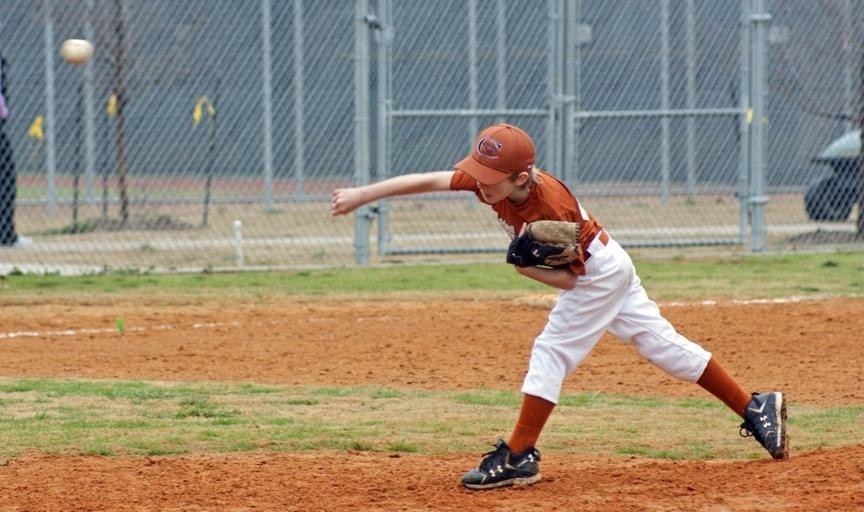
[453,124,536,186]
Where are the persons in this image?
[331,121,789,489]
[0,51,33,248]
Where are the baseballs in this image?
[59,39,93,64]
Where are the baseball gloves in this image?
[506,221,580,268]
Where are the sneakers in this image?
[744,391,788,459]
[462,439,542,489]
